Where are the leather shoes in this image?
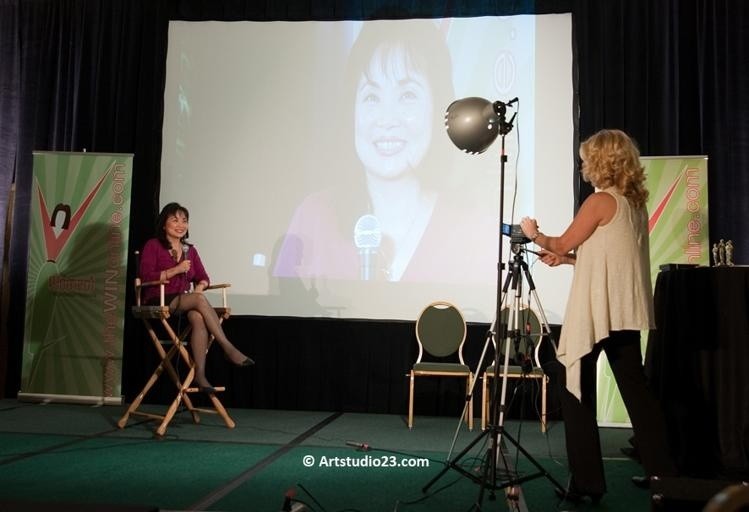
[227,350,256,368]
[192,376,216,393]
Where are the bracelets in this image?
[164,270,169,280]
[559,254,570,264]
[198,282,206,288]
[532,232,543,244]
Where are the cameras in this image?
[502,223,527,237]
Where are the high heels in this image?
[555,482,606,507]
[631,471,667,490]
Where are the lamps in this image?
[421,93,575,510]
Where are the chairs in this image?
[405,300,553,438]
[110,249,236,434]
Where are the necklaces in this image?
[166,237,184,259]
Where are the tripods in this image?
[423,135,568,504]
[443,254,558,468]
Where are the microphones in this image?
[181,243,190,280]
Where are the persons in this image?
[712,238,734,267]
[518,128,674,508]
[271,19,486,282]
[137,202,257,394]
